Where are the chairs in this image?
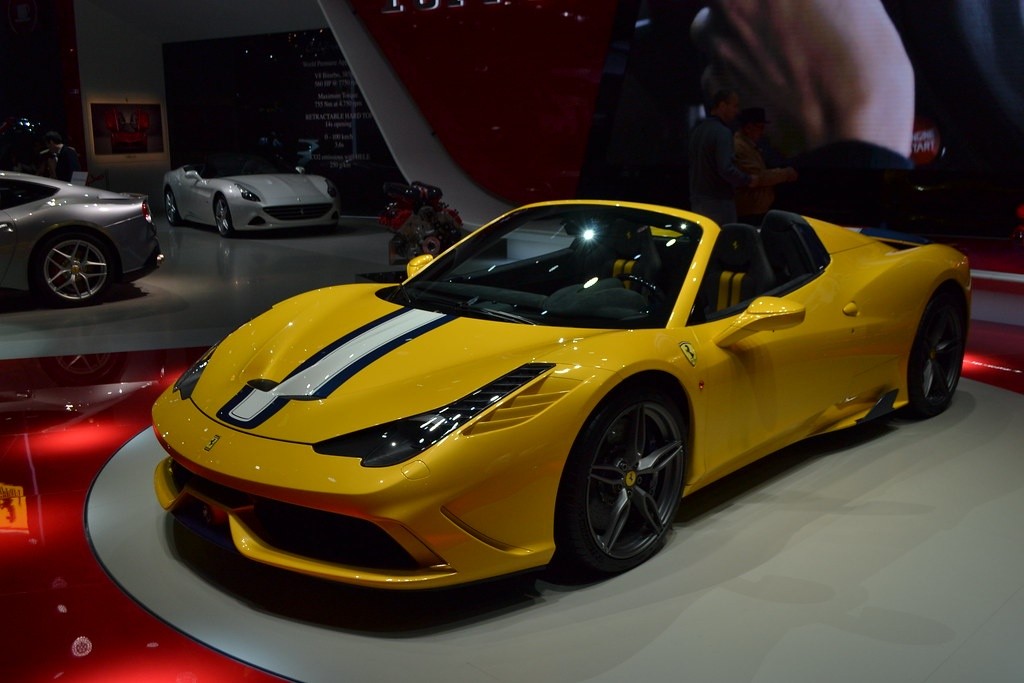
[583,224,664,299]
[702,221,773,319]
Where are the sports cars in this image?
[151,198,974,598]
[160,158,344,236]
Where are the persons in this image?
[0,131,82,183]
[732,107,798,227]
[688,89,759,226]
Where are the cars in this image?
[0,172,163,307]
